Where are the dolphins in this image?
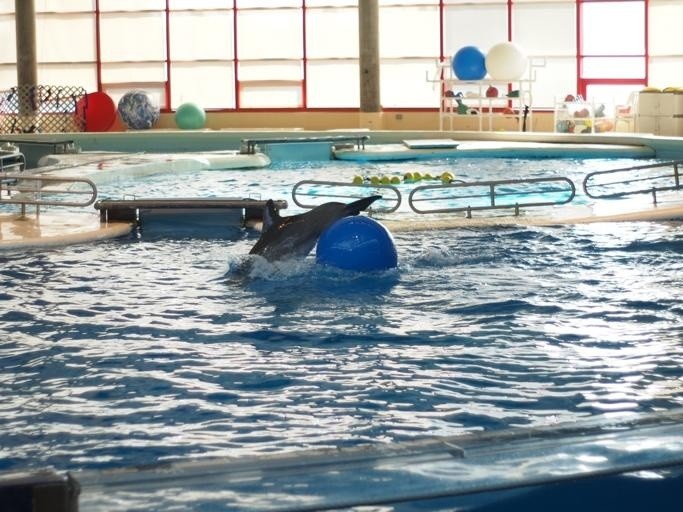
[248,195,382,262]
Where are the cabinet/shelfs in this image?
[426,57,547,133]
